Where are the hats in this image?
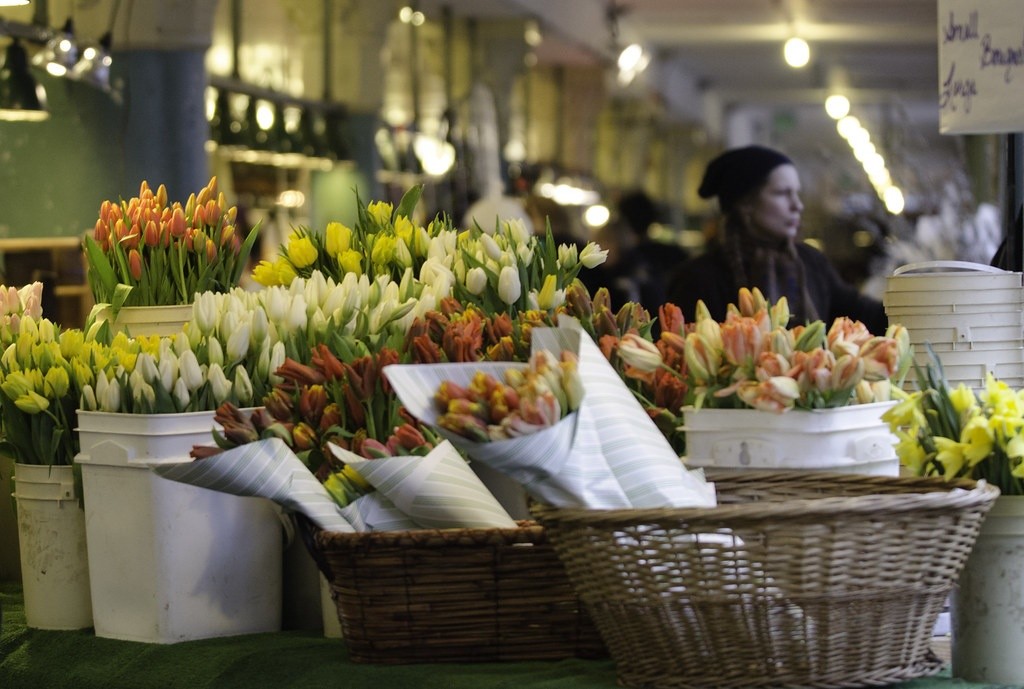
[698,146,794,214]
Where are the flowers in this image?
[0,175,1024,688]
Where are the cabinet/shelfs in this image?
[0,237,95,329]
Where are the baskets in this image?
[303,519,613,666]
[526,464,1001,689]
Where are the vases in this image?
[949,495,1024,687]
[319,570,342,638]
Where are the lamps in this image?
[603,4,644,69]
[32,0,124,92]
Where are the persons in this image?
[593,144,889,337]
